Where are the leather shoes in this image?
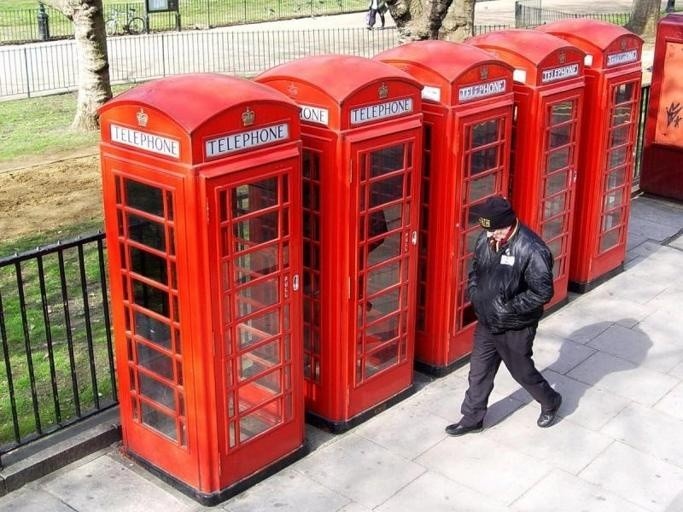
[445,419,483,437]
[537,392,562,427]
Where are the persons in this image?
[366,0,388,30]
[445,194,563,439]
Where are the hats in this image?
[477,198,516,230]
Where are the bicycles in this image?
[104,8,145,37]
